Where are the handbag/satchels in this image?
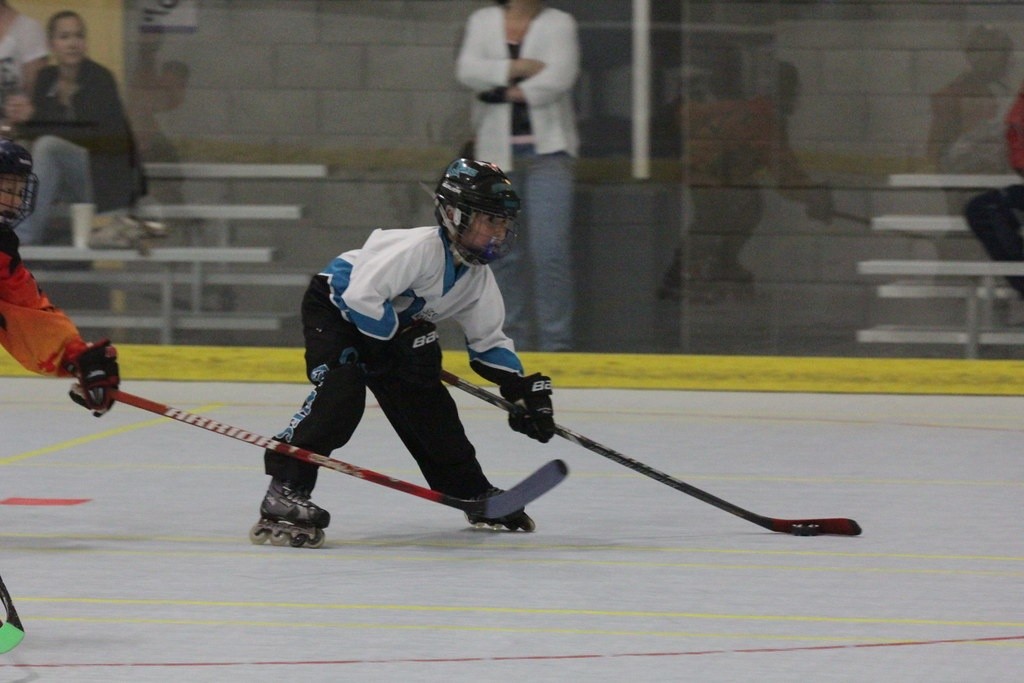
[86,210,168,257]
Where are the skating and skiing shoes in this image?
[464,486,536,532]
[248,473,330,549]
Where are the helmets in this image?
[434,158,522,265]
[0,135,39,230]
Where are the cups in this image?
[70,202,96,249]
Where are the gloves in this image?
[500,372,555,444]
[66,338,120,418]
[384,316,443,394]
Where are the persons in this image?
[1,0,149,245]
[454,0,581,353]
[964,78,1023,295]
[130,15,190,203]
[658,56,834,304]
[250,157,557,547]
[927,26,1013,295]
[1,143,121,654]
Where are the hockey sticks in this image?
[105,384,569,522]
[443,369,863,539]
[0,573,26,655]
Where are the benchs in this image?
[855,169,1024,361]
[19,159,325,346]
